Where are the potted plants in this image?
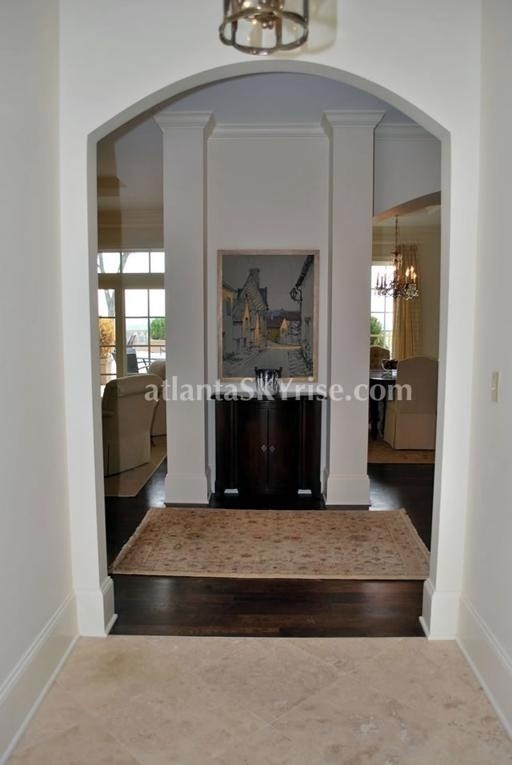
[101,317,116,385]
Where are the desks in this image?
[370,368,399,437]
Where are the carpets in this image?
[106,507,431,579]
[106,446,166,497]
[367,435,436,466]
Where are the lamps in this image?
[376,215,422,302]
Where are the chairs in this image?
[370,346,390,368]
[101,359,166,476]
[384,357,437,451]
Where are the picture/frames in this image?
[216,249,320,383]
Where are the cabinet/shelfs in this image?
[210,397,323,509]
